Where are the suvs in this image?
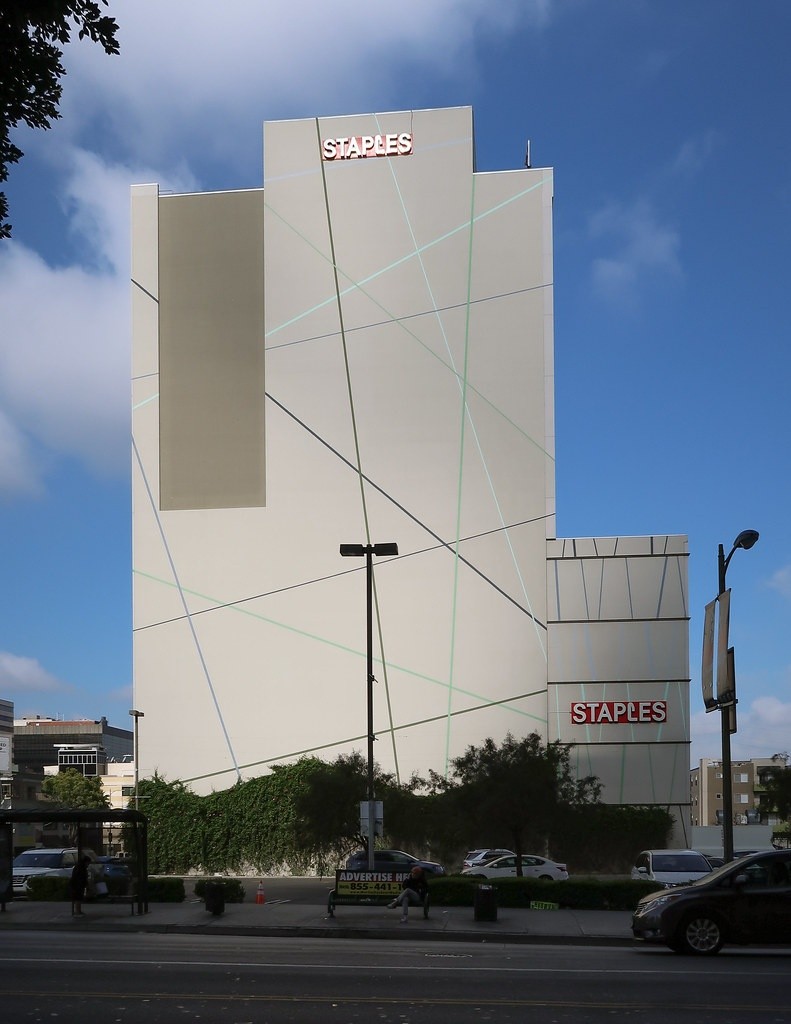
[345,849,445,876]
[13,848,102,894]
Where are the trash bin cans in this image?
[206,881,226,916]
[474,885,497,922]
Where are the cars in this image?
[706,858,765,874]
[461,854,569,882]
[463,850,531,866]
[97,856,132,882]
[632,849,712,886]
[630,850,791,958]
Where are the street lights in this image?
[129,710,144,828]
[340,543,400,869]
[719,529,759,857]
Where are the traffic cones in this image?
[256,880,266,904]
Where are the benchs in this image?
[328,869,430,921]
[72,894,139,916]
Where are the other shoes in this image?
[388,899,398,909]
[401,915,408,922]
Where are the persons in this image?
[72,856,90,918]
[386,866,428,922]
